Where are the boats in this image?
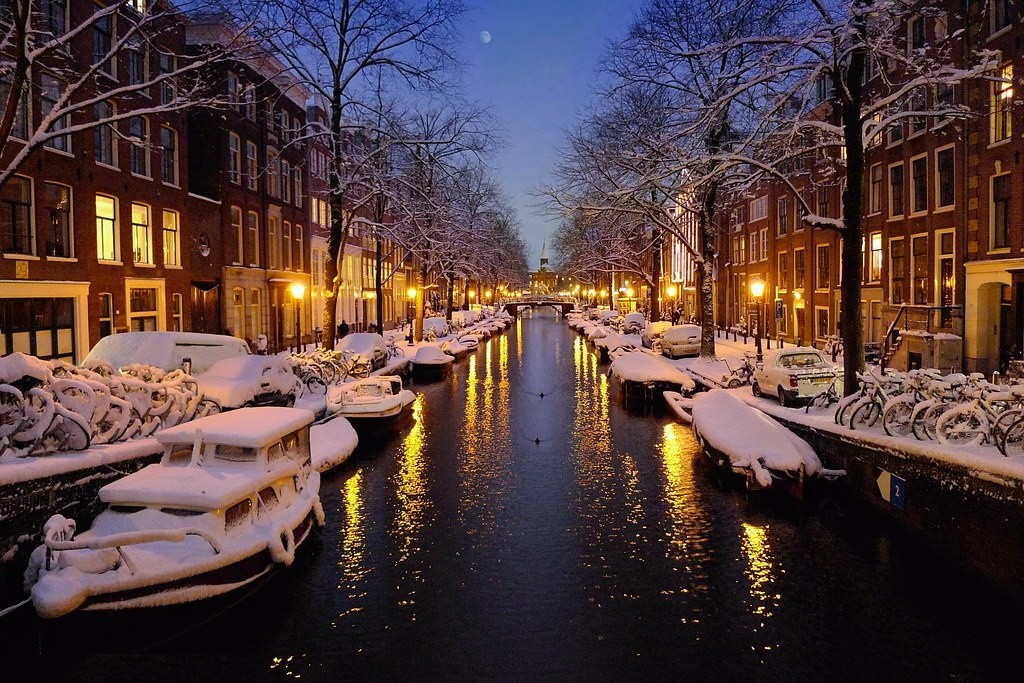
[29,408,323,617]
[567,313,683,391]
[310,316,515,473]
[692,387,822,481]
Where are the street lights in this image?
[749,278,766,370]
[407,289,417,346]
[666,286,676,326]
[469,292,474,310]
[291,283,305,354]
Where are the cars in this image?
[589,309,646,334]
[641,321,677,348]
[335,305,496,374]
[660,324,701,360]
[751,345,844,407]
[193,355,297,417]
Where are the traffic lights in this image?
[572,285,633,311]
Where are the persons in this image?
[678,310,684,325]
[338,320,350,339]
[432,289,443,312]
[223,328,233,338]
[675,307,683,326]
[256,334,267,355]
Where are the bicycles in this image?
[805,367,1024,457]
[386,335,404,361]
[286,347,370,397]
[0,352,223,458]
